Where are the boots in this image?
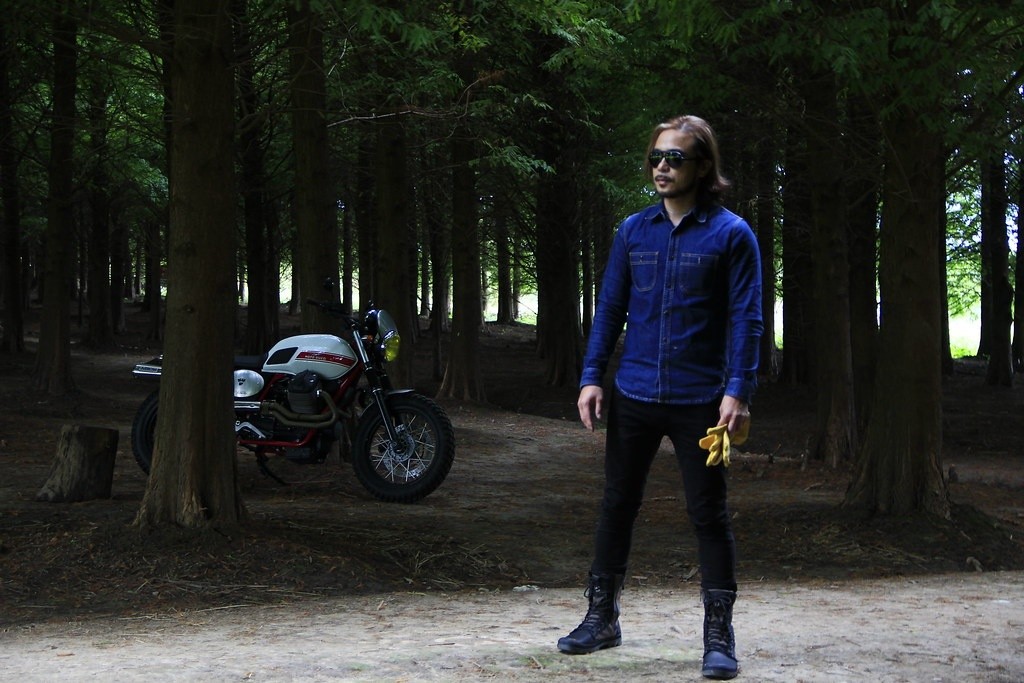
[698,587,739,679]
[556,571,627,655]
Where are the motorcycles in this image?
[128,298,456,503]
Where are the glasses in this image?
[648,148,693,168]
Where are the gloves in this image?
[698,411,752,467]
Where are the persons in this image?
[557,115,764,679]
